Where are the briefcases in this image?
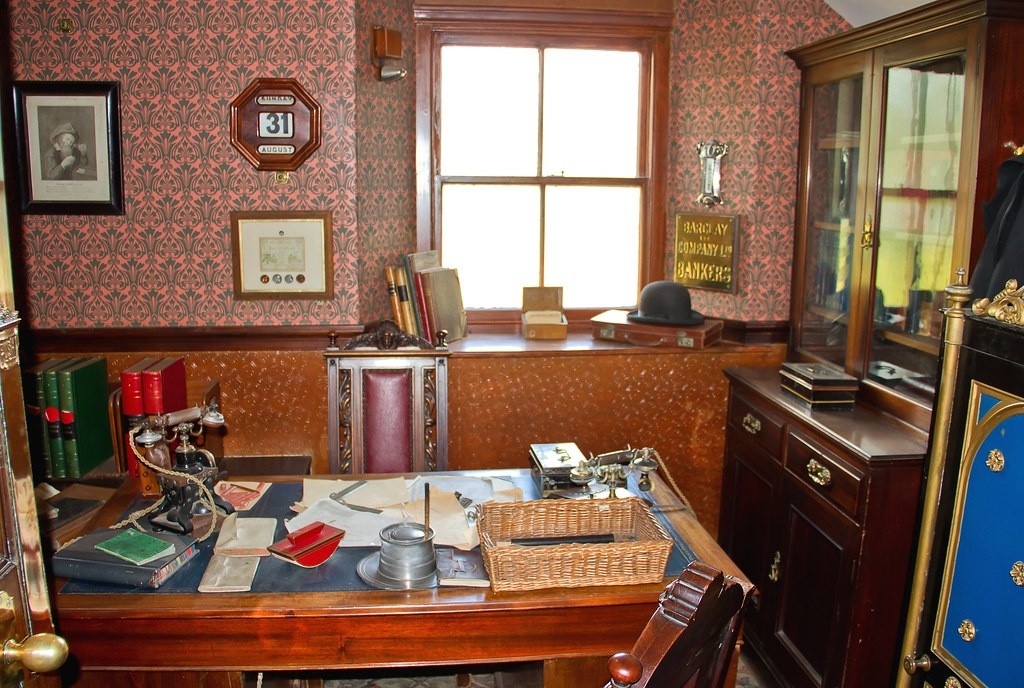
[777,362,859,413]
[867,360,931,390]
[527,442,589,498]
[589,309,724,350]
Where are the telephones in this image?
[569,444,659,509]
[134,405,236,542]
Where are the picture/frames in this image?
[228,210,335,300]
[12,81,124,214]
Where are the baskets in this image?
[475,495,675,593]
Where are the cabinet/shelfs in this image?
[718,0,1024,687]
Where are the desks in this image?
[33,465,757,688]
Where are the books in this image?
[50,527,200,589]
[93,528,176,567]
[37,495,106,531]
[21,352,191,482]
[384,249,471,348]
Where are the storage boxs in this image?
[521,286,567,339]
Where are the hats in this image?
[628,280,704,326]
[49,122,79,146]
[831,284,904,329]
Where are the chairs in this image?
[323,320,453,476]
